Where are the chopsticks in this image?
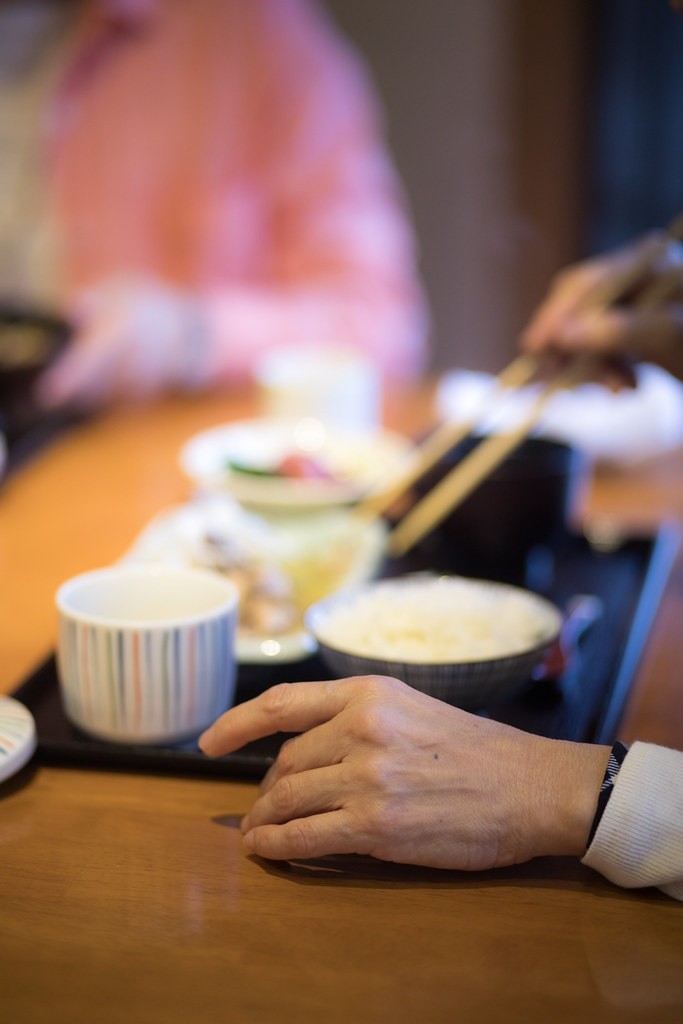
[356,216,683,555]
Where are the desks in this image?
[0,377,683,1024]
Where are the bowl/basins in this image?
[180,417,421,506]
[310,573,564,713]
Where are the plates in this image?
[116,497,388,664]
[0,694,37,783]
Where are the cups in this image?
[53,566,238,755]
[254,347,377,427]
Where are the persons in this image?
[197,234,679,899]
